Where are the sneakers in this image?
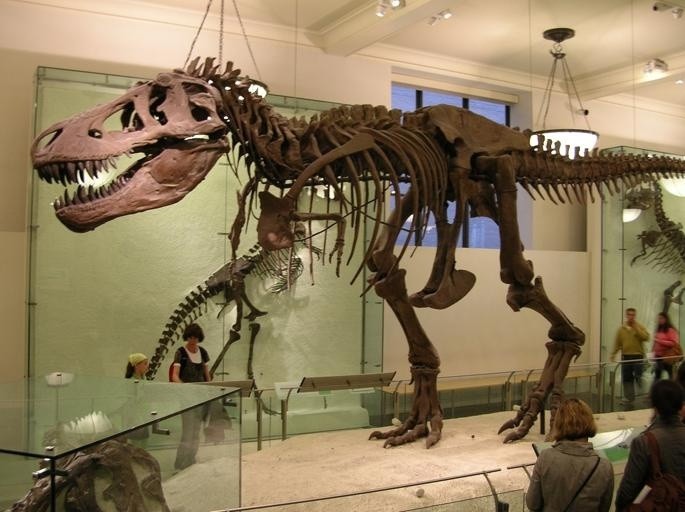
[622,397,630,402]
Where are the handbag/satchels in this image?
[663,345,683,365]
[642,353,651,373]
[626,474,684,511]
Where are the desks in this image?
[379,367,600,424]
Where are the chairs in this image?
[274,379,370,437]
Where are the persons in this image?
[526,398,614,512]
[610,308,650,403]
[615,381,685,512]
[169,323,210,469]
[651,312,679,381]
[125,353,151,448]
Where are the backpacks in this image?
[170,347,187,382]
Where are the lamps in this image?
[527,26,601,158]
[183,1,267,105]
